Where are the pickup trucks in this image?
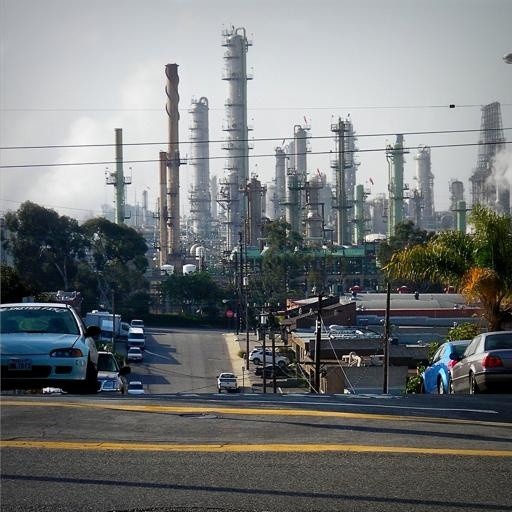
[215,372,239,394]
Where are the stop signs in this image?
[226,310,234,318]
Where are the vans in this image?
[84,309,146,396]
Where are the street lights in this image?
[329,324,388,394]
[259,310,268,394]
[234,338,266,394]
[223,299,249,371]
[276,309,320,394]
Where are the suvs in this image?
[249,348,289,367]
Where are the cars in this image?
[1,302,102,395]
[42,350,131,396]
[420,338,472,393]
[448,331,512,394]
